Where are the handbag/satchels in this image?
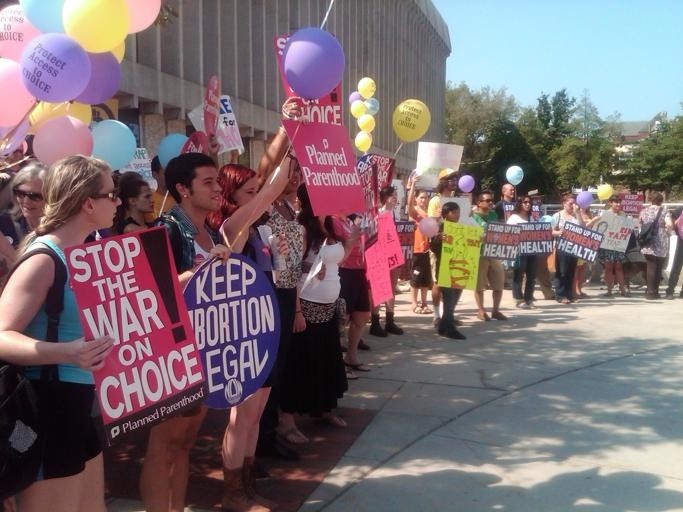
[0,365,45,501]
[638,220,657,248]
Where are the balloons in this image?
[281,28,346,100]
[385,99,432,172]
[458,175,475,193]
[349,76,383,153]
[0,0,190,171]
[505,166,524,186]
[419,218,439,237]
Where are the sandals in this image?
[413,307,425,314]
[421,305,432,313]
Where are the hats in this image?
[439,168,458,180]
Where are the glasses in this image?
[522,201,531,203]
[479,199,493,202]
[13,189,43,201]
[91,191,118,200]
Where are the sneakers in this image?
[492,312,507,320]
[479,312,490,321]
[439,325,466,339]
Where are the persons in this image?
[523,189,556,301]
[429,202,467,340]
[506,195,537,309]
[0,95,404,510]
[467,189,509,322]
[427,168,463,328]
[540,184,683,320]
[406,172,433,314]
[492,184,519,290]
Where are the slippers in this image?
[343,360,370,371]
[345,368,360,379]
[318,415,347,428]
[275,427,309,449]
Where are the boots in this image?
[386,312,403,335]
[243,461,279,510]
[370,314,387,337]
[221,466,271,512]
